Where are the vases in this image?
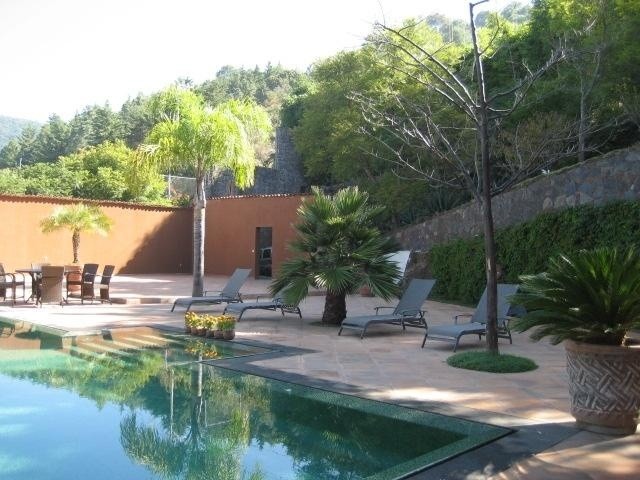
[183,326,236,340]
[358,281,374,297]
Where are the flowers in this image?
[183,311,238,330]
[183,338,218,359]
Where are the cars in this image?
[256,247,272,276]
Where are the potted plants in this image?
[38,196,113,293]
[500,240,640,436]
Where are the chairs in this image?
[420,282,521,353]
[0,261,116,306]
[170,267,253,315]
[337,277,437,340]
[221,276,310,324]
